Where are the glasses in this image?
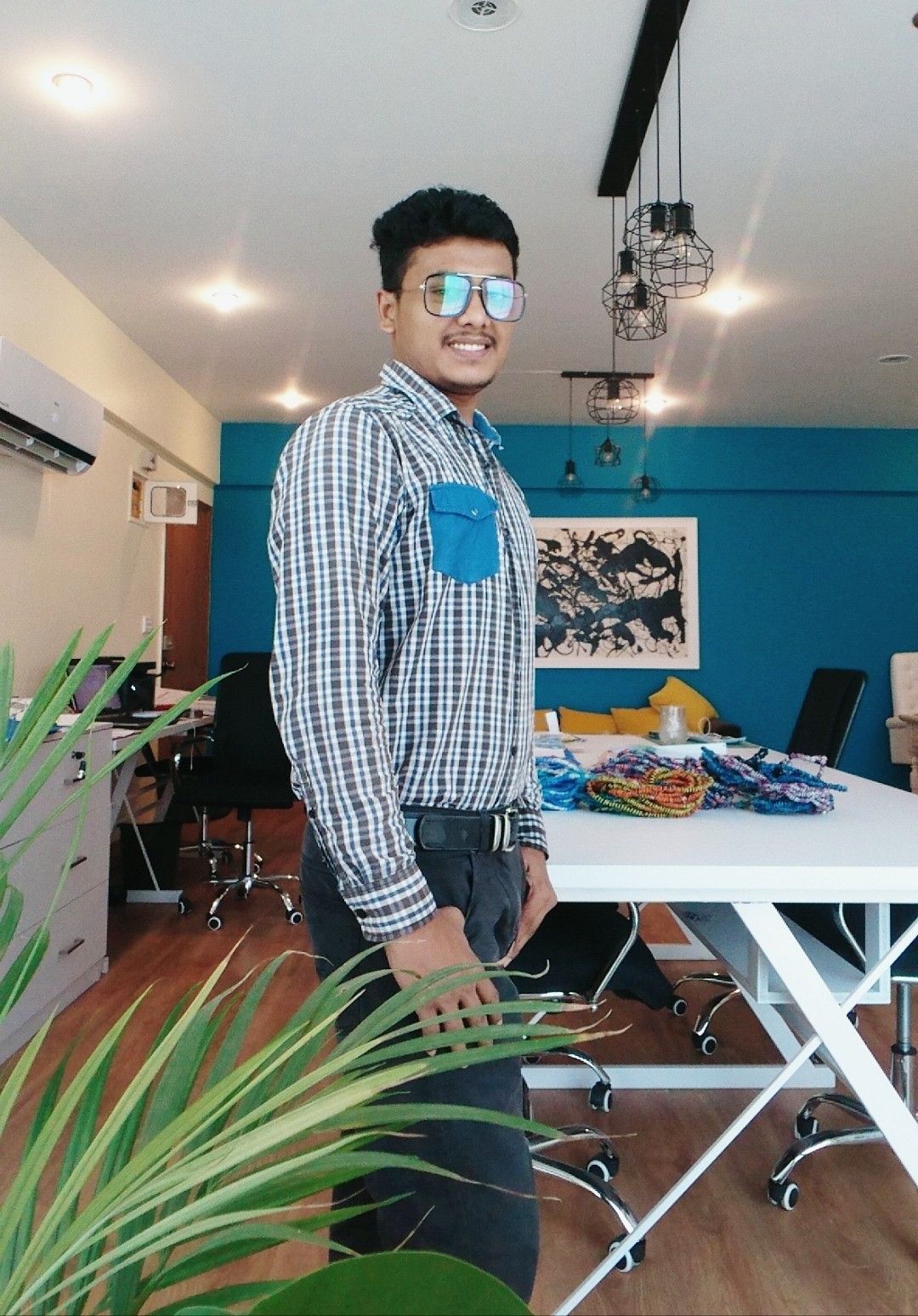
[393,272,528,323]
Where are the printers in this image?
[97,656,158,714]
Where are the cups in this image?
[659,705,689,745]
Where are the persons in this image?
[267,185,556,1306]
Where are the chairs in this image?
[170,652,306,932]
[509,902,670,1273]
[885,652,918,795]
[767,900,918,1210]
[670,668,870,1057]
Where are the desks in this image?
[521,733,918,1316]
[112,716,214,905]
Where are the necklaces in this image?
[534,747,848,819]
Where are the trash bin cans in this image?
[117,821,182,891]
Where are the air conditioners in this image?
[0,336,104,476]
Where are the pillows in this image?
[609,706,662,733]
[535,709,552,731]
[558,704,616,733]
[650,676,718,732]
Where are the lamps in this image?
[646,32,715,301]
[611,154,668,339]
[602,189,645,320]
[555,372,656,506]
[585,197,641,425]
[622,97,690,269]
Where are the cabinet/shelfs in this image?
[0,713,113,1064]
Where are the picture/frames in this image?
[526,517,700,670]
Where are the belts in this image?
[404,807,520,855]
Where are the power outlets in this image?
[144,618,154,635]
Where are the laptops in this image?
[64,658,180,730]
